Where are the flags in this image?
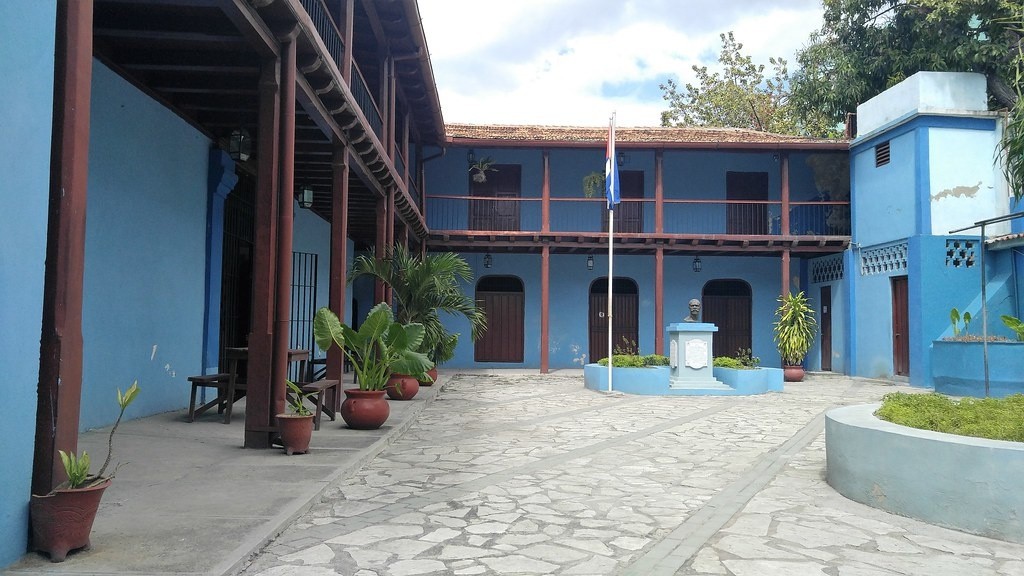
[605,123,620,210]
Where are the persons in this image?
[683,299,701,323]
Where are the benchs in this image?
[189,374,339,430]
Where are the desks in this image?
[225,346,310,423]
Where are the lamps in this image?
[586,254,594,270]
[483,251,492,268]
[231,122,250,163]
[693,255,702,273]
[618,151,624,166]
[467,149,475,163]
[298,178,313,208]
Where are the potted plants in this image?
[318,301,409,428]
[273,380,321,456]
[368,303,435,401]
[341,242,490,385]
[30,380,143,562]
[774,292,818,382]
